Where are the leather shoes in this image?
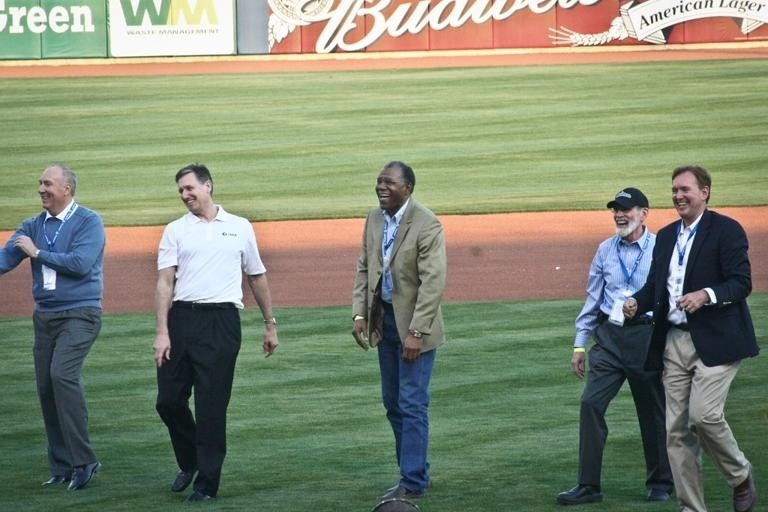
[66,457,102,493]
[732,462,756,512]
[646,488,671,503]
[39,475,68,484]
[167,468,197,491]
[555,481,603,505]
[376,482,425,500]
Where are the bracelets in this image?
[33,249,41,258]
[573,347,585,353]
[410,331,423,339]
[354,315,365,320]
[264,317,276,325]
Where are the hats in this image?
[607,184,650,210]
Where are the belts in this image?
[171,300,235,312]
[600,315,653,326]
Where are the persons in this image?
[0,164,107,493]
[557,185,674,506]
[350,160,452,501]
[622,165,761,512]
[152,163,280,502]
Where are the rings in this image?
[688,305,691,309]
